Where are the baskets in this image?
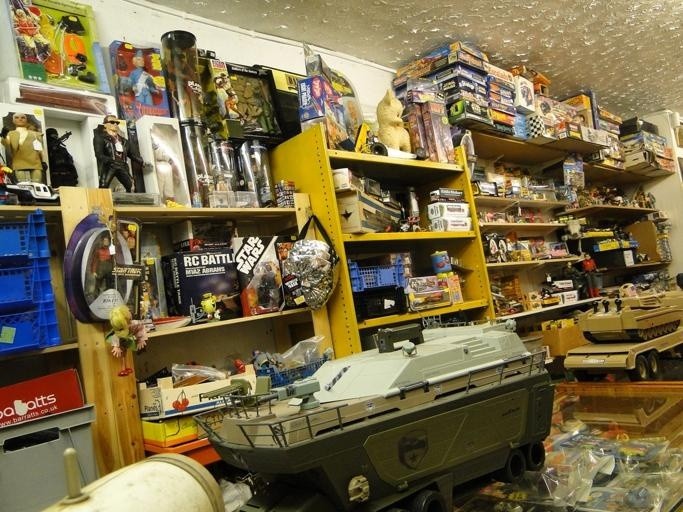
[0,208,62,356]
[255,357,328,389]
[348,256,408,320]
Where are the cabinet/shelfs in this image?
[0,120,664,481]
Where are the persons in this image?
[602,300,610,313]
[615,299,622,311]
[564,261,585,289]
[126,54,160,106]
[1,113,180,205]
[592,301,598,313]
[90,229,285,381]
[168,54,273,193]
[583,254,599,297]
